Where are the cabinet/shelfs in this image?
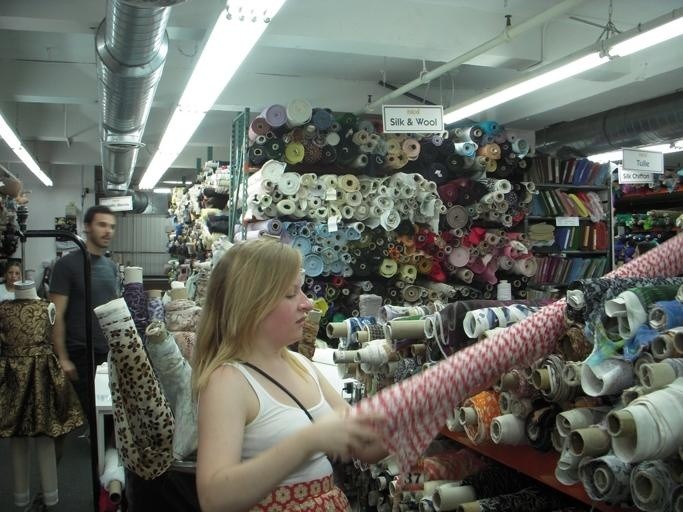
[528,162,615,288]
[616,170,683,265]
[230,108,528,308]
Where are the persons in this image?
[0,260,22,301]
[45,204,118,510]
[189,238,395,511]
[0,278,84,507]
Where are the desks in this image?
[94,360,114,476]
